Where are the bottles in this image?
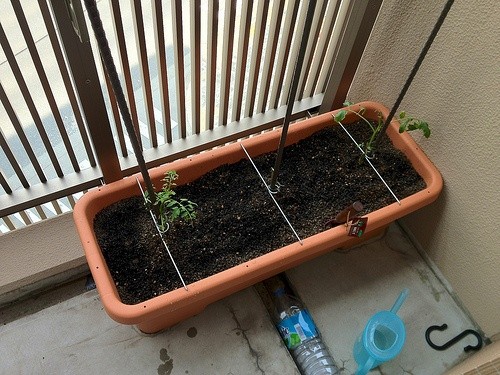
[270,284,337,375]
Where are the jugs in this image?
[351,286,412,375]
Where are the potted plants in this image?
[72,101,443,335]
